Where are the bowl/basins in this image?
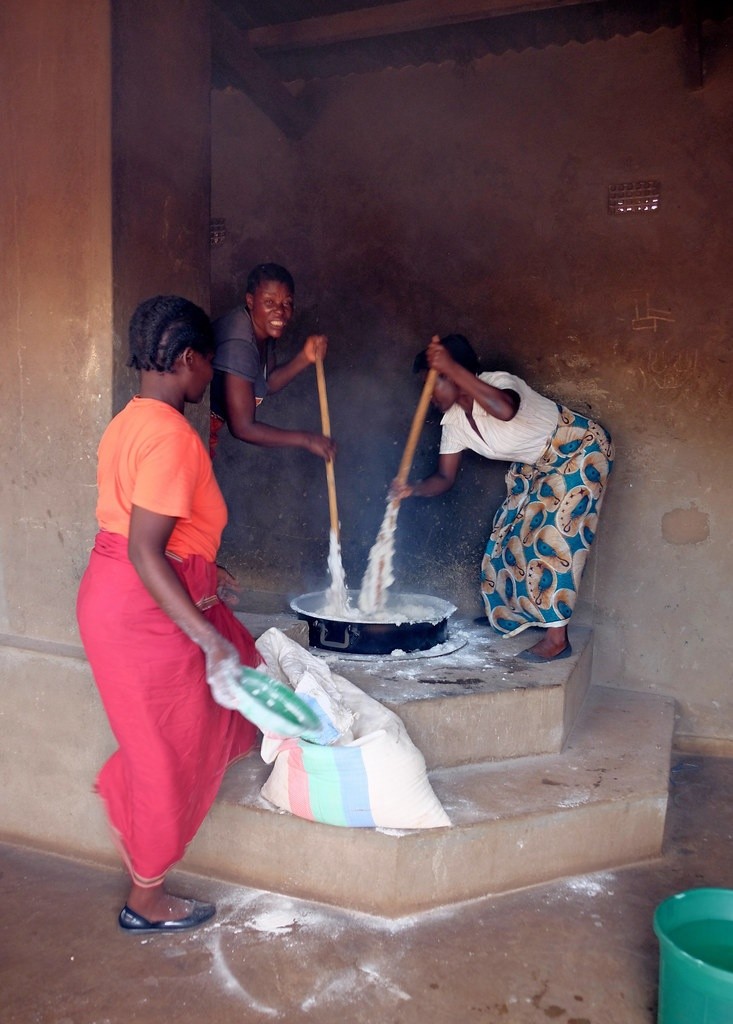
[227,666,321,737]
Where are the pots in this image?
[290,590,457,655]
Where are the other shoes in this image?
[473,616,491,627]
[515,644,572,663]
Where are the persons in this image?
[208,262,338,462]
[383,333,613,667]
[73,294,266,931]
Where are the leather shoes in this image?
[119,899,216,934]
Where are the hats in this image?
[414,333,481,375]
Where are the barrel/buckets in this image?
[652,886,733,1024]
